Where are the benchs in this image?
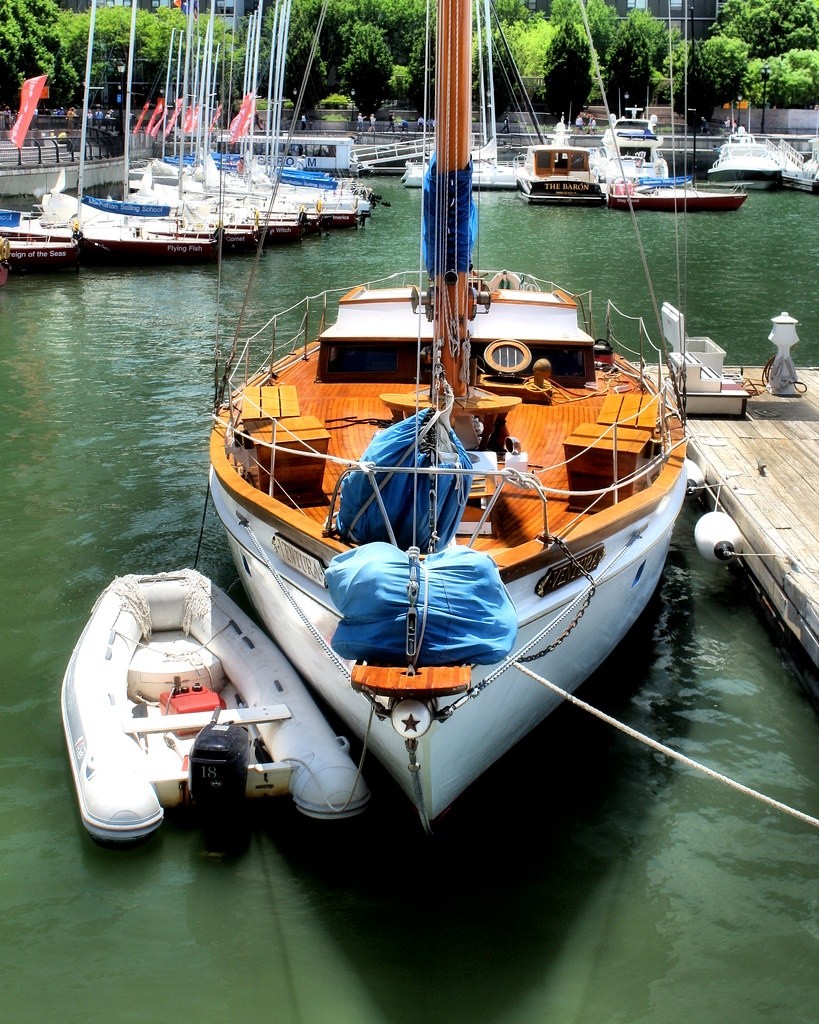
[125,705,293,737]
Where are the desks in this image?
[377,394,523,449]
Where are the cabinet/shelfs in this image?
[454,451,500,537]
[562,390,657,513]
[242,385,332,503]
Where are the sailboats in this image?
[0,0,396,274]
[203,1,694,844]
[402,0,545,191]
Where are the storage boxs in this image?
[661,301,727,377]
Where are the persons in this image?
[386,115,395,132]
[418,116,424,132]
[501,115,510,133]
[299,145,303,156]
[45,107,65,115]
[725,117,731,132]
[237,157,244,172]
[105,109,116,130]
[576,114,596,135]
[66,107,77,128]
[701,117,710,135]
[399,120,408,131]
[0,100,44,131]
[358,113,366,131]
[95,108,103,124]
[287,149,292,156]
[255,111,263,130]
[88,109,94,126]
[734,120,737,128]
[368,114,376,132]
[301,113,307,130]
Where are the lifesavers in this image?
[490,272,523,290]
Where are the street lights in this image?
[350,89,356,121]
[292,88,298,111]
[116,62,125,136]
[761,62,769,133]
[737,93,743,126]
[624,92,629,119]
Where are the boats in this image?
[708,126,787,191]
[512,142,608,209]
[57,567,369,852]
[588,87,670,181]
[607,172,746,212]
